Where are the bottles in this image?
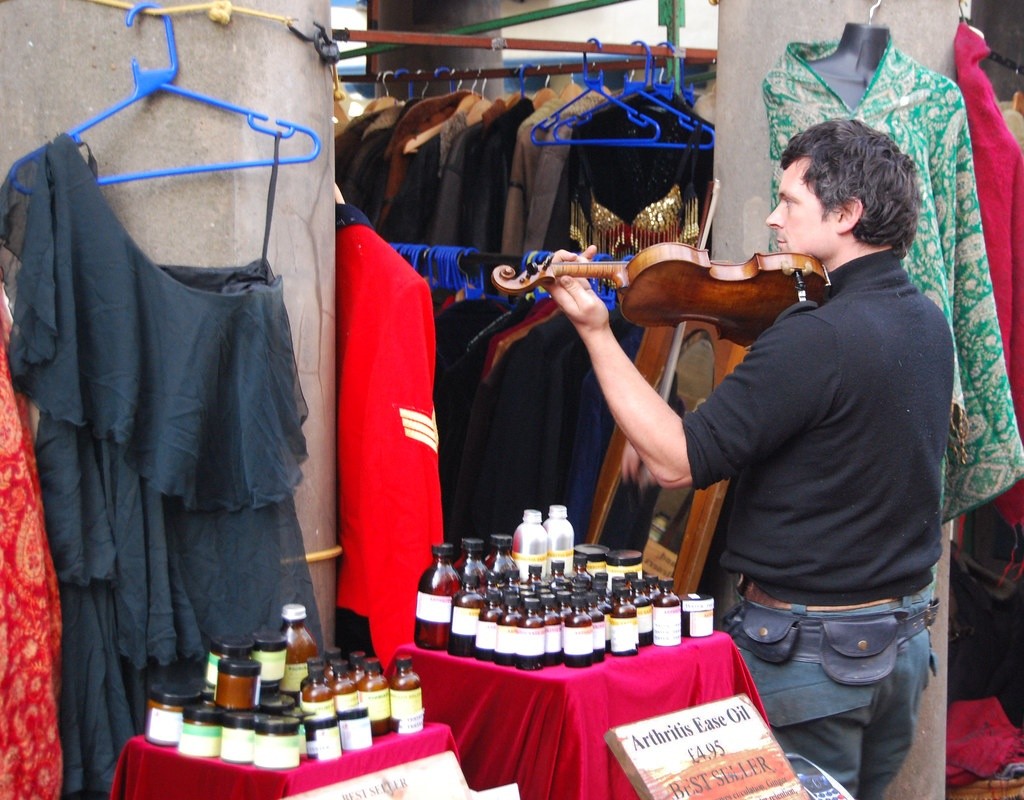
[144,603,425,769]
[413,544,462,651]
[448,505,715,670]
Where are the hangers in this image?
[342,20,722,156]
[8,1,322,194]
[762,0,1024,128]
[376,238,635,321]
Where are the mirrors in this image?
[587,316,770,592]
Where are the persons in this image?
[763,21,1024,526]
[543,117,957,800]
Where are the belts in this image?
[736,574,899,611]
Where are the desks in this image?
[110,625,776,800]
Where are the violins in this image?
[494,239,831,346]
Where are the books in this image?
[282,694,808,800]
